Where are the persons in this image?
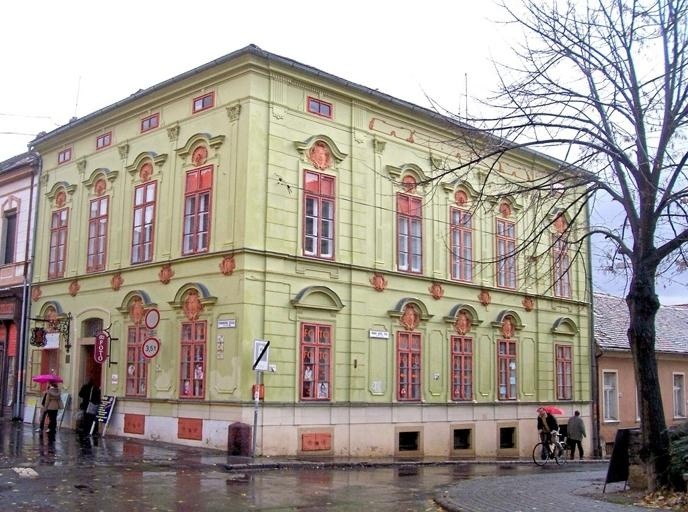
[34,384,50,433]
[79,377,101,432]
[534,407,564,457]
[45,381,62,433]
[566,410,587,460]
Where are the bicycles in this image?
[533,431,568,466]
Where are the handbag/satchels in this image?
[87,402,98,415]
[58,399,65,410]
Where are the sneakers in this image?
[46,428,51,432]
[35,428,42,432]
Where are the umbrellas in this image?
[539,406,561,414]
[32,375,63,383]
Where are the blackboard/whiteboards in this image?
[95,396,116,422]
